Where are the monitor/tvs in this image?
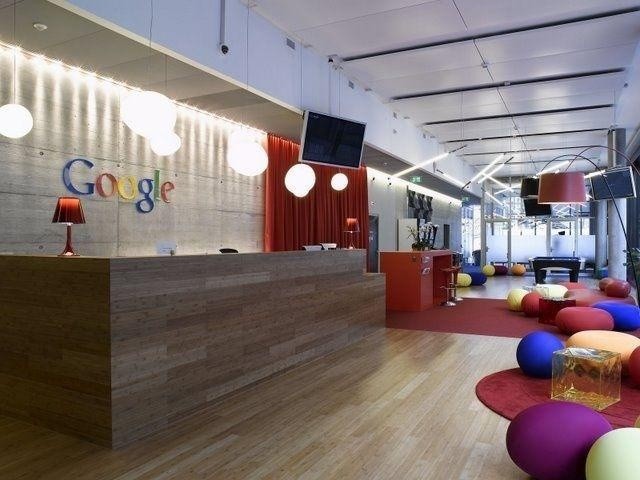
[321,243,337,250]
[524,198,552,216]
[298,109,368,170]
[589,166,637,201]
[302,246,322,253]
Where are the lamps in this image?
[436,169,469,189]
[460,152,506,191]
[331,70,348,191]
[537,145,640,204]
[482,173,516,194]
[285,39,316,197]
[584,169,606,179]
[481,187,504,207]
[228,0,269,176]
[51,197,86,256]
[122,0,178,140]
[477,156,513,185]
[341,218,360,250]
[386,145,467,180]
[535,160,571,176]
[0,0,33,138]
[521,154,640,307]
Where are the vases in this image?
[406,222,439,251]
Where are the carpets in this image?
[564,287,635,304]
[475,368,640,430]
[384,298,640,341]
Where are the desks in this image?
[380,250,452,312]
